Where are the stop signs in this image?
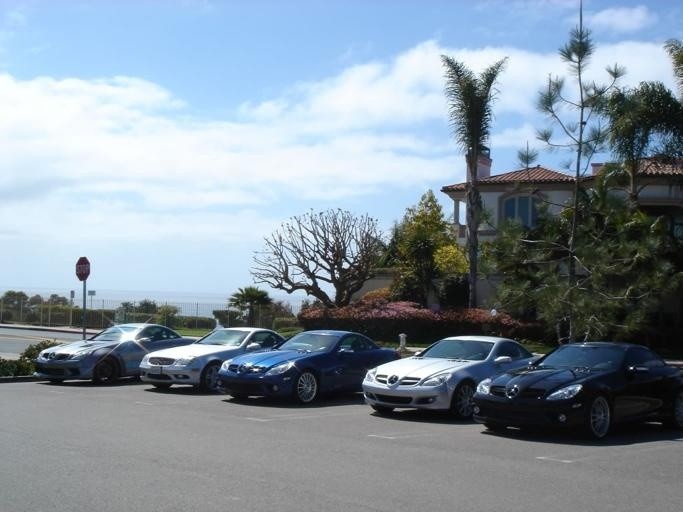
[75,257,90,281]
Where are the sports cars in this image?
[359,333,545,424]
[467,340,683,442]
[138,326,287,392]
[214,328,402,407]
[32,322,204,385]
